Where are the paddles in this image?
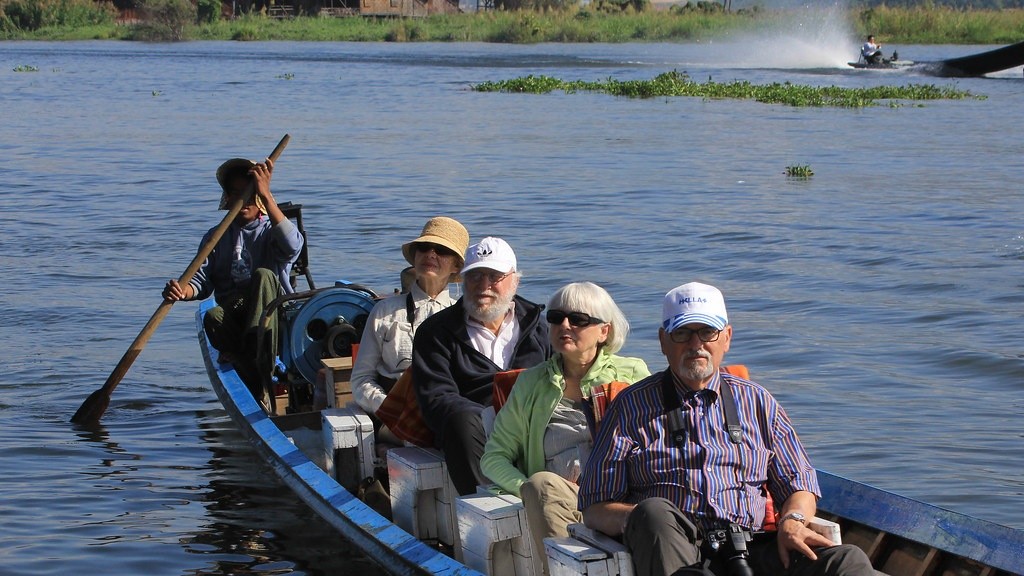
[70,131,292,425]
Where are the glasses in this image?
[417,243,454,256]
[468,269,514,284]
[670,326,724,343]
[546,310,606,327]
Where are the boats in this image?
[195,202,1024,576]
[847,42,1024,77]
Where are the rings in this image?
[263,167,267,170]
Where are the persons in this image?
[480,281,652,576]
[162,158,305,400]
[413,236,555,496]
[864,35,882,64]
[350,217,469,447]
[576,282,874,576]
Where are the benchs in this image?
[317,356,840,576]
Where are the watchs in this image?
[778,512,805,527]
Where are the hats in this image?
[216,158,262,210]
[664,282,728,334]
[403,217,469,276]
[460,237,517,275]
